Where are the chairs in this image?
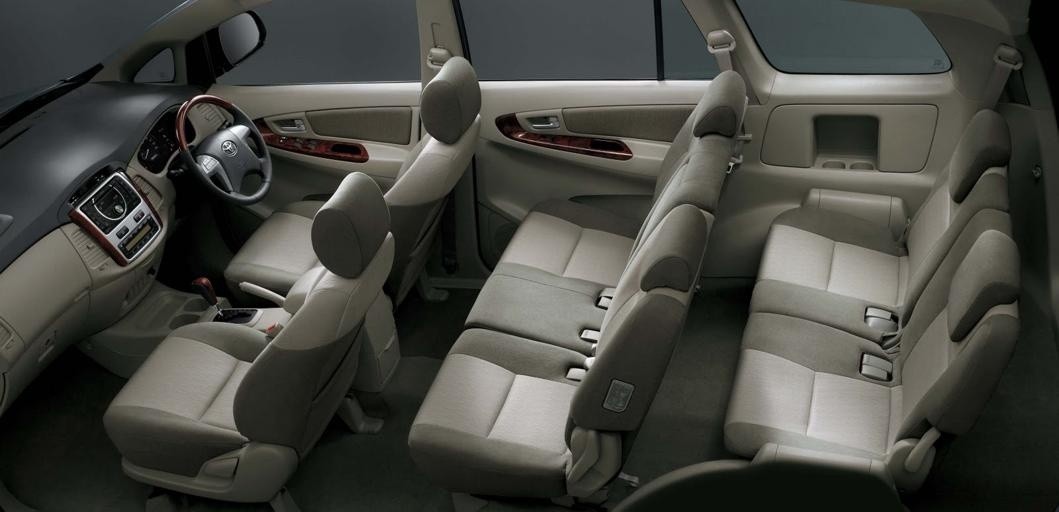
[102,54,1028,507]
